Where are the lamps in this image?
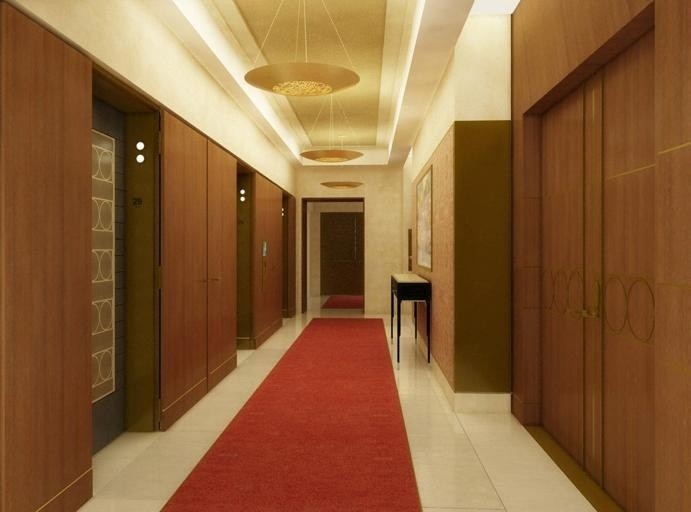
[244,0,361,96]
[319,164,365,188]
[298,93,363,162]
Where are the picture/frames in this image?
[415,164,433,271]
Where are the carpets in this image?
[152,316,422,511]
[319,294,363,309]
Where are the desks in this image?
[388,272,431,365]
